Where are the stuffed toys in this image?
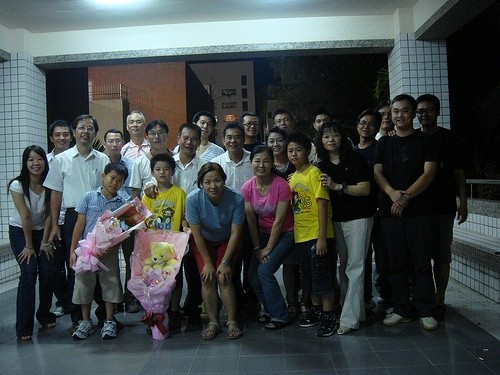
[142,242,179,275]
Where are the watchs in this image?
[222,260,232,266]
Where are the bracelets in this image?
[42,243,50,246]
[253,245,260,250]
[339,182,346,194]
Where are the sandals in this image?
[201,321,221,339]
[266,321,289,330]
[18,336,32,341]
[226,319,242,339]
[259,314,271,323]
[46,323,56,328]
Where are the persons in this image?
[388,95,467,311]
[46,101,394,331]
[240,144,294,329]
[140,153,192,335]
[69,163,128,339]
[286,121,373,335]
[285,133,340,337]
[42,115,125,333]
[6,145,56,342]
[374,94,439,330]
[185,163,246,340]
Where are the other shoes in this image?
[125,296,140,313]
[114,302,123,314]
[288,303,298,312]
[337,326,351,334]
[383,313,412,326]
[183,314,203,330]
[53,307,65,316]
[146,313,181,333]
[70,321,79,331]
[419,316,438,330]
[300,301,311,315]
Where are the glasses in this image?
[244,123,260,127]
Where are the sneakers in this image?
[316,313,338,336]
[101,319,119,339]
[72,319,96,339]
[299,310,322,327]
